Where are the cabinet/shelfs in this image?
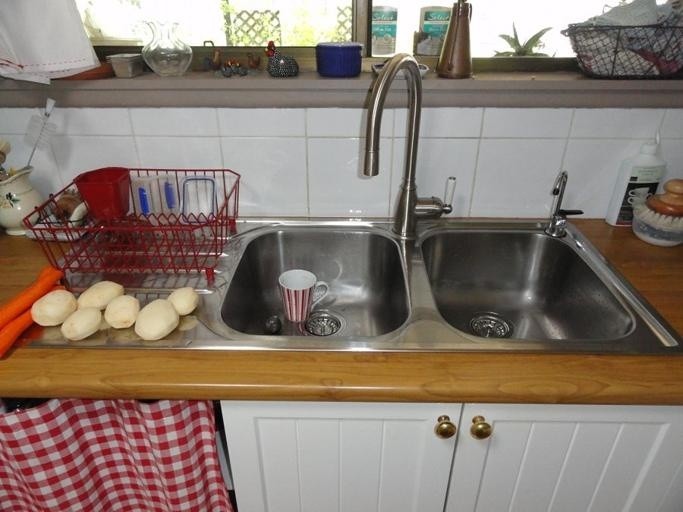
[220,398,682,510]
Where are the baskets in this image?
[561,22,683,81]
[22,165,243,287]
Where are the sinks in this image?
[196,220,410,341]
[416,224,638,342]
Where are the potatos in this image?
[30,280,199,341]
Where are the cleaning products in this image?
[604,128,667,228]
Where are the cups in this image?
[0,166,47,235]
[279,269,329,324]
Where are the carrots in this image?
[0,266,67,358]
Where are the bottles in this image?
[436,0,473,78]
[141,16,193,77]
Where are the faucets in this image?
[363,52,458,235]
